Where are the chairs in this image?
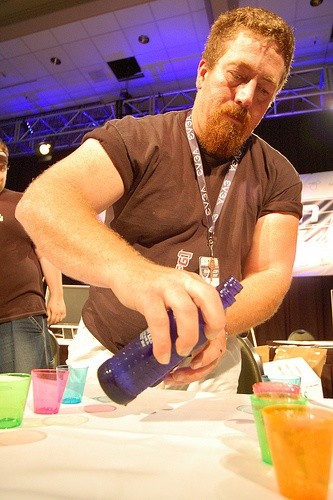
[288,329,314,343]
[45,284,89,345]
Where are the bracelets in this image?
[225,330,228,341]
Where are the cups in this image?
[0,373,31,429]
[251,376,307,466]
[262,404,333,500]
[29,369,70,414]
[56,365,88,404]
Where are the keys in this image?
[209,260,215,280]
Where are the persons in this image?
[0,142,67,375]
[14,7,302,394]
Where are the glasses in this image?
[0,165,9,172]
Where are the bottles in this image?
[97,275,244,406]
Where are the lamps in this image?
[36,139,55,158]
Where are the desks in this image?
[273,340,333,348]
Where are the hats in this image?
[0,142,8,163]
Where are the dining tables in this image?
[1,376,333,500]
